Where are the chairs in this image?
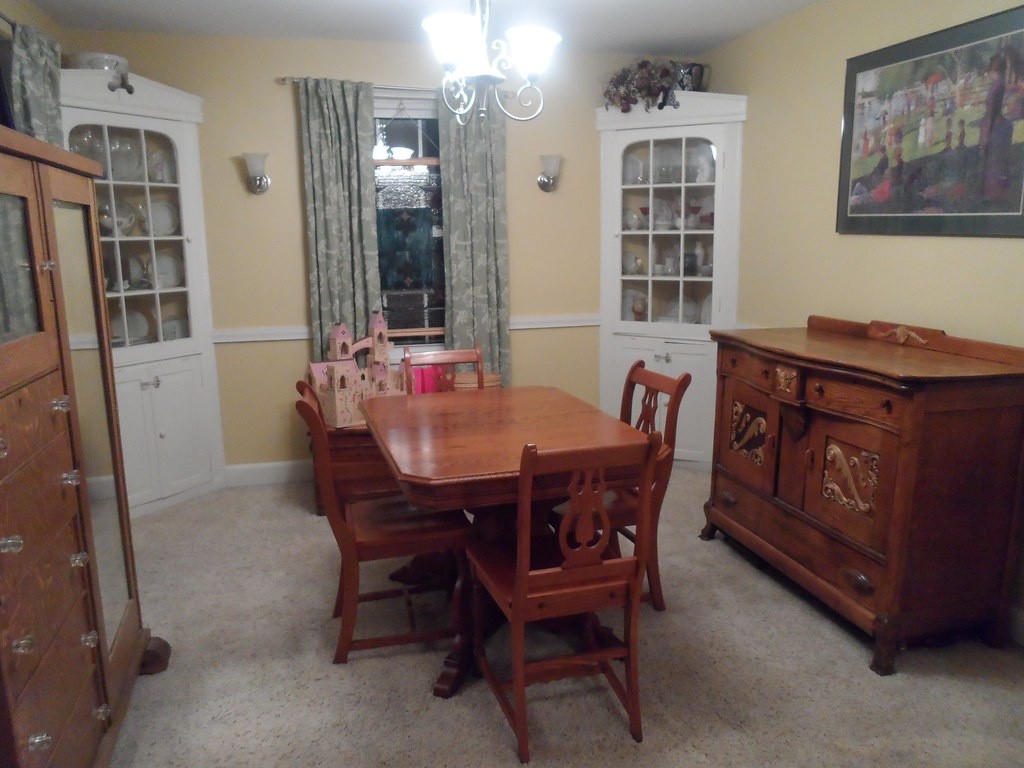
[465,430,662,763]
[294,343,485,665]
[550,360,692,612]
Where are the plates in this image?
[145,201,180,235]
[147,252,184,287]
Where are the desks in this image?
[354,385,651,699]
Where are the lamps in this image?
[241,153,271,196]
[536,154,560,192]
[421,0,563,127]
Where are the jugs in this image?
[676,63,704,91]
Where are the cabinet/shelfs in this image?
[0,123,172,768]
[54,67,226,520]
[695,315,1023,676]
[596,89,746,461]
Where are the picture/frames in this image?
[835,3,1024,236]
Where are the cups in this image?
[633,298,645,321]
